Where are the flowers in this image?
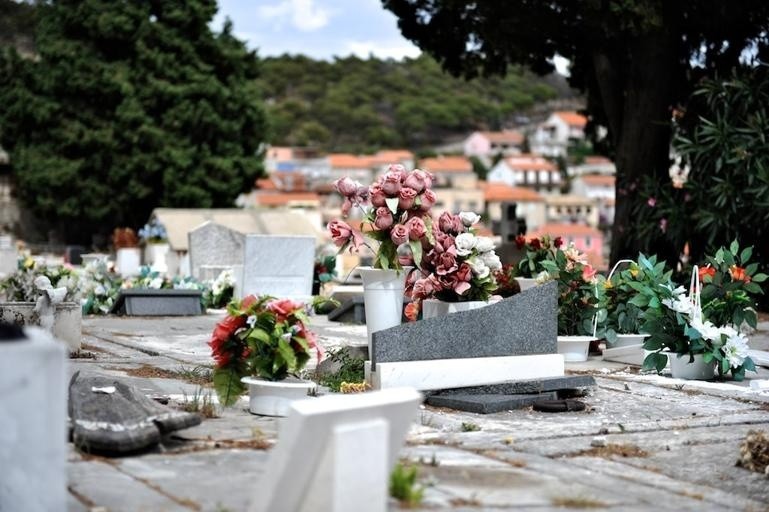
[604,251,767,366]
[208,295,321,381]
[327,160,518,301]
[12,238,234,311]
[513,227,597,282]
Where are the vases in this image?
[355,261,413,356]
[664,350,716,381]
[608,330,651,352]
[514,275,545,293]
[557,330,596,362]
[421,293,498,318]
[240,376,316,416]
